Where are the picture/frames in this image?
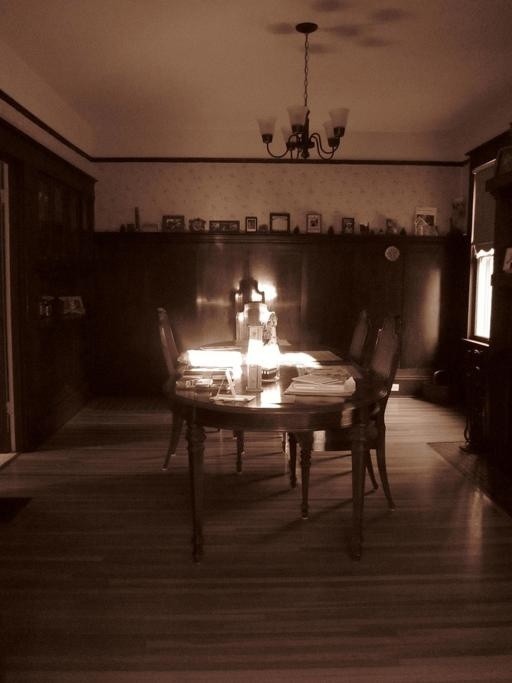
[159,207,356,234]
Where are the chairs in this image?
[288,311,402,520]
[154,307,245,476]
[281,309,375,471]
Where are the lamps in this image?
[256,21,351,162]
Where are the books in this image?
[176,350,245,382]
[284,374,356,397]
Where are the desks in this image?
[161,336,390,568]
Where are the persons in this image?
[345,223,352,233]
[416,215,428,227]
[311,219,316,226]
